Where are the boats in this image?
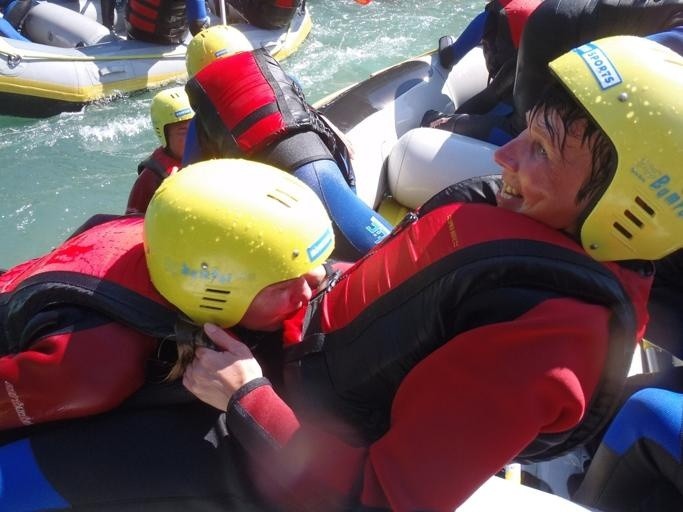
[0,0,315,116]
[303,31,683,512]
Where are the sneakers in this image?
[439,35,454,69]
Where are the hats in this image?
[150,85,196,149]
[185,25,254,79]
[547,33,681,263]
[141,158,336,330]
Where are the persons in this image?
[2,1,683,510]
